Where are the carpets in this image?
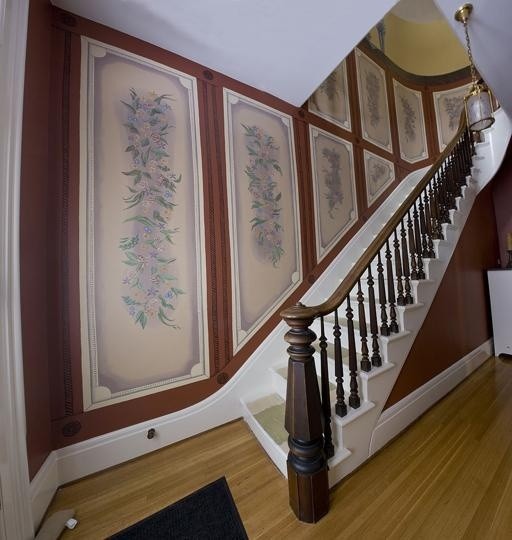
[100,473,253,538]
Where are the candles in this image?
[505,231,510,253]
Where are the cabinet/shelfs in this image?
[485,266,512,359]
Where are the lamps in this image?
[452,1,497,134]
